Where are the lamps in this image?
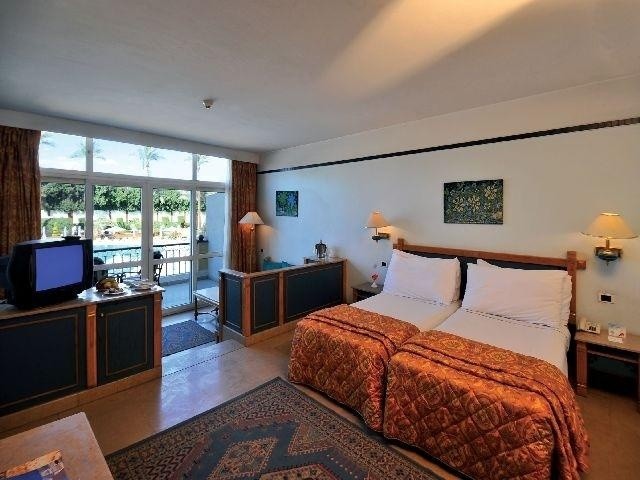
[579,213,639,267]
[238,211,264,274]
[361,211,392,241]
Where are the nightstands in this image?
[351,283,383,303]
[575,329,640,412]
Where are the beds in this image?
[287,239,585,480]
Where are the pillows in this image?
[476,259,572,325]
[454,259,461,302]
[462,261,567,328]
[382,248,456,306]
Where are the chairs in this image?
[121,250,164,283]
[93,258,118,285]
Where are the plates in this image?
[98,280,156,295]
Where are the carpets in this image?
[103,377,447,480]
[161,320,216,358]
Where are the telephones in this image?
[577,317,601,334]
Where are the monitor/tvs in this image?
[7,239,93,308]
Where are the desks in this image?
[0,410,115,480]
[191,286,220,321]
